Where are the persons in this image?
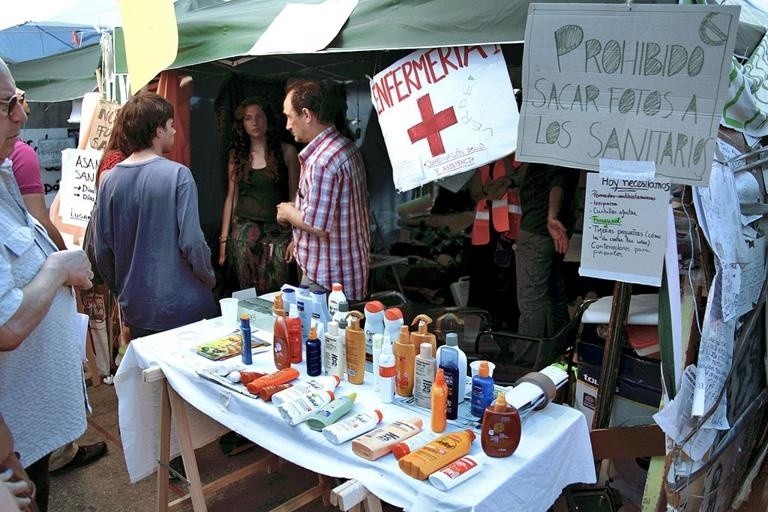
[275,76,369,306]
[5,90,108,476]
[1,59,96,512]
[470,160,522,327]
[1,469,31,512]
[87,92,263,487]
[96,114,127,193]
[509,163,582,367]
[215,96,301,293]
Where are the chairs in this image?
[456,300,596,407]
[369,209,410,296]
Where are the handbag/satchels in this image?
[567,487,622,512]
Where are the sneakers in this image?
[219,432,256,456]
[169,458,183,479]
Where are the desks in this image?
[129,315,584,512]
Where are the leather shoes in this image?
[49,442,106,474]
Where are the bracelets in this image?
[481,185,488,196]
[219,241,226,244]
[218,235,229,239]
[507,175,515,189]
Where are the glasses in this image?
[0,88,25,116]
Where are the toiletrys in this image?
[240,283,521,491]
